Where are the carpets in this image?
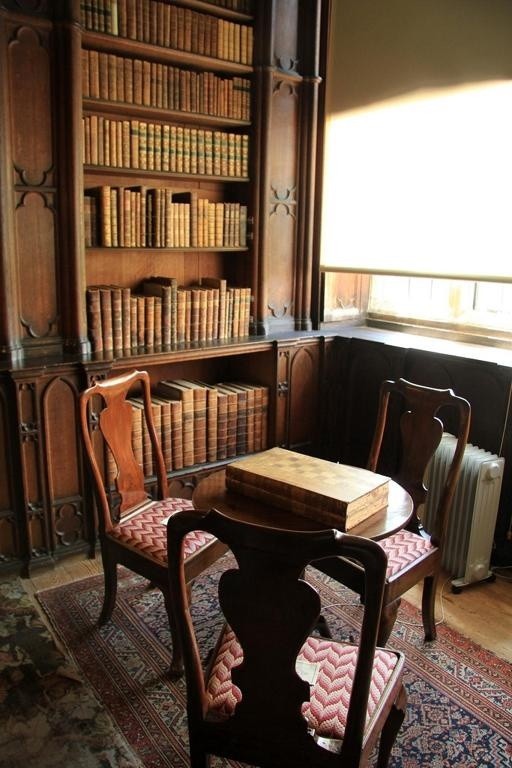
[31,551,512,766]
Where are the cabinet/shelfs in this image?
[57,1,282,485]
[88,341,278,558]
[278,338,512,466]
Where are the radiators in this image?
[418,429,507,596]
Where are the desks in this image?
[193,468,414,541]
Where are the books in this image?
[85,276,251,353]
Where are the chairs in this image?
[167,508,407,768]
[80,369,230,672]
[300,377,472,646]
